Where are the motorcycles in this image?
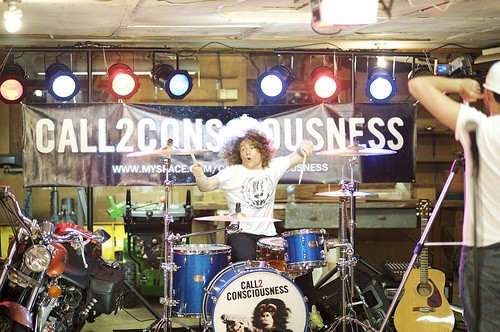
[0,184,128,332]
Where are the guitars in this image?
[394,198,455,332]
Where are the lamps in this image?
[0,46,437,106]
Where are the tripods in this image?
[327,238,378,332]
[154,220,240,332]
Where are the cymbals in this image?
[194,212,282,222]
[315,188,370,196]
[315,144,398,156]
[127,146,214,158]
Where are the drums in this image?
[283,228,328,269]
[255,237,285,270]
[202,261,309,332]
[168,243,232,318]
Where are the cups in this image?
[114,251,124,259]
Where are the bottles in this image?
[287,185,296,204]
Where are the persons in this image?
[409,61,500,332]
[192,130,313,265]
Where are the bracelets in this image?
[297,148,303,158]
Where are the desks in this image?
[272,197,464,271]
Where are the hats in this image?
[483,61,500,95]
